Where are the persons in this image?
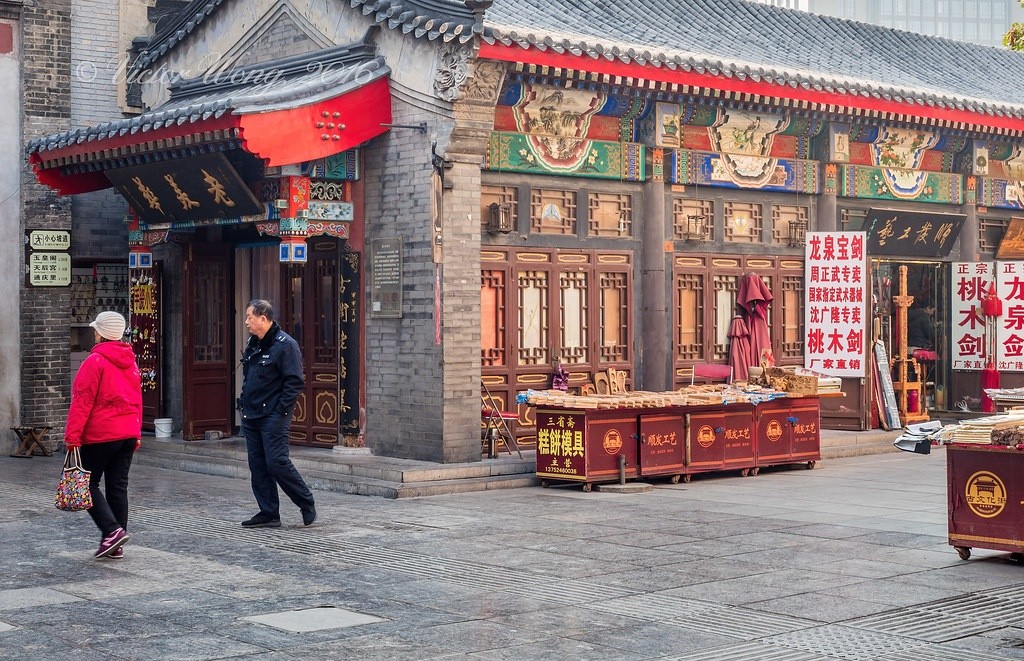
[908,297,934,379]
[237,299,317,529]
[64,311,142,558]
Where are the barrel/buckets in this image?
[154,418,174,437]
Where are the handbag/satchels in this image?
[55,447,93,511]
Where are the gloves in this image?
[135,440,140,450]
[65,442,81,450]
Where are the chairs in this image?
[480,379,523,459]
[691,364,734,384]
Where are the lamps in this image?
[786,158,808,248]
[487,129,513,236]
[684,148,707,246]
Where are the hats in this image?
[89,311,125,340]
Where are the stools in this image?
[8,426,53,458]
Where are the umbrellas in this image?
[737,272,773,367]
[728,315,754,381]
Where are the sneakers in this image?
[300,504,317,526]
[242,511,282,527]
[108,546,123,558]
[95,527,130,558]
